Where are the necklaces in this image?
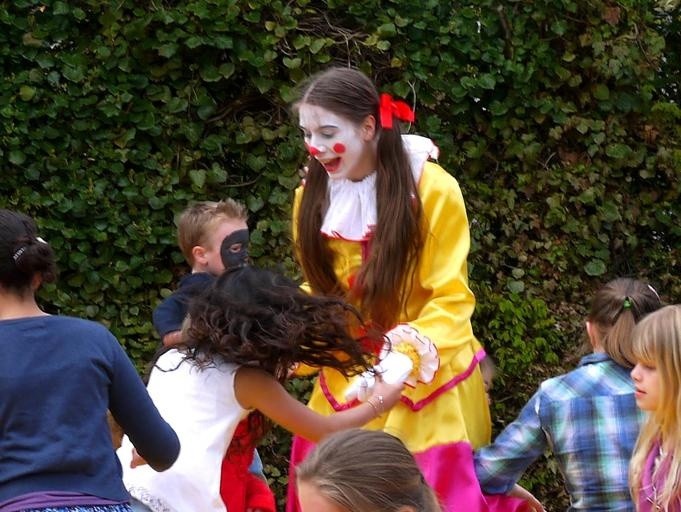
[654,457,662,467]
[660,445,666,457]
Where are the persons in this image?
[281,66,536,511]
[0,204,179,512]
[625,299,679,512]
[473,274,665,512]
[149,194,298,483]
[115,263,411,512]
[292,426,446,512]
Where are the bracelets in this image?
[373,394,384,411]
[364,397,379,416]
